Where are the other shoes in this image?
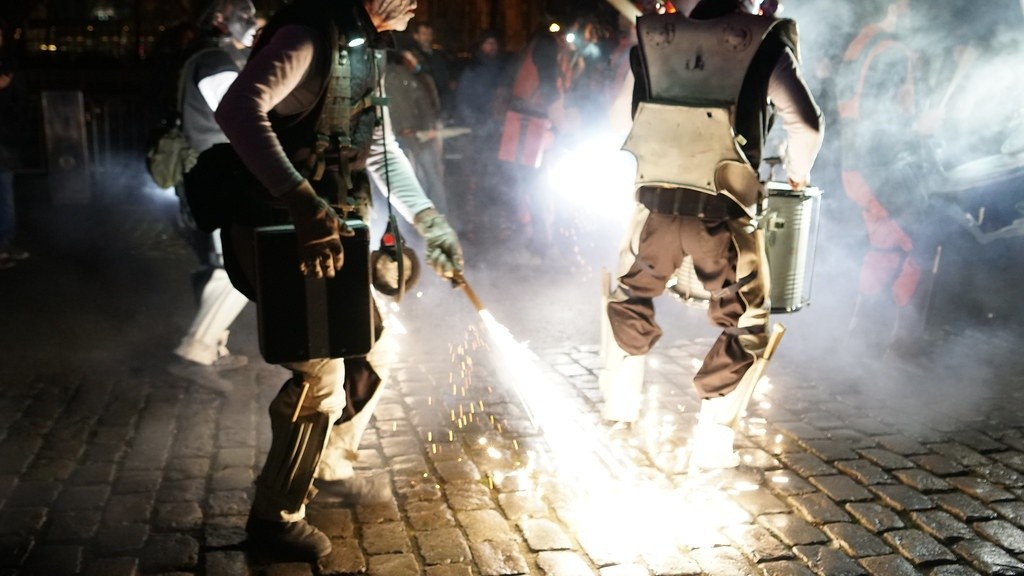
[247,518,335,559]
[164,353,251,395]
[308,475,396,511]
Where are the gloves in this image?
[277,178,356,283]
[417,212,465,290]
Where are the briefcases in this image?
[497,110,550,169]
[666,181,824,315]
[255,220,375,365]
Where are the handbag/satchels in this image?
[147,125,199,189]
[181,142,237,237]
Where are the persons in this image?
[152,0,1022,564]
